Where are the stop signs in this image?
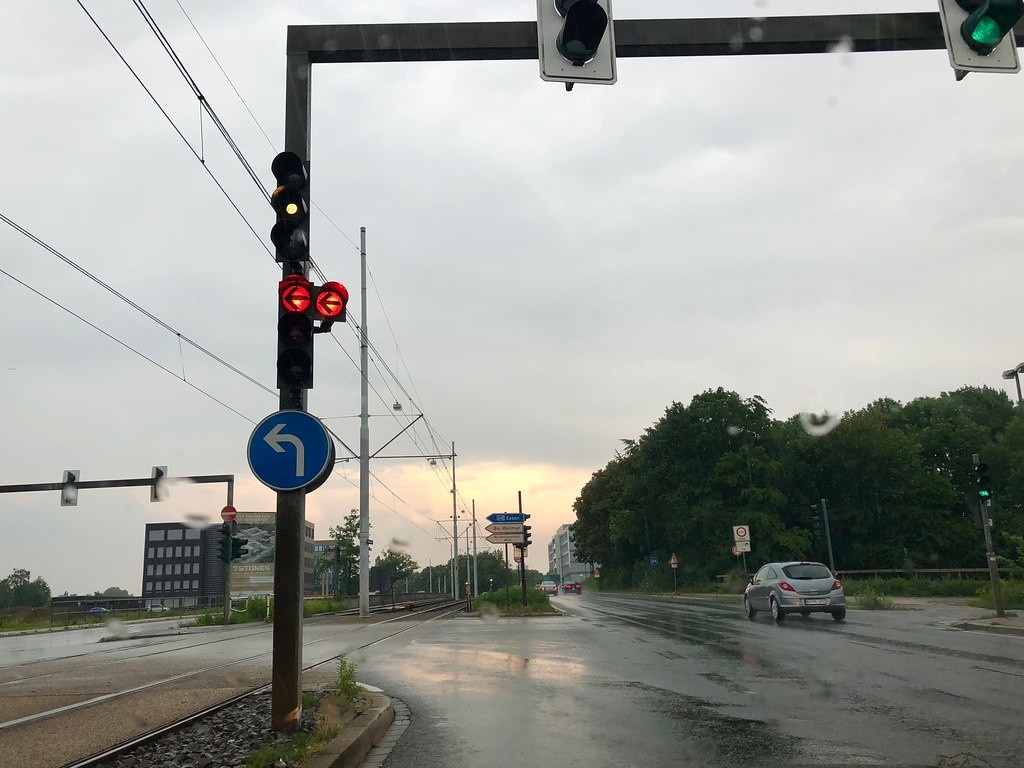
[221,506,238,522]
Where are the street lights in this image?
[1001,362,1024,410]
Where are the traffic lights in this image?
[274,278,317,389]
[231,537,249,561]
[808,500,825,539]
[536,0,620,87]
[489,577,495,592]
[972,463,994,503]
[313,282,352,322]
[268,150,312,267]
[522,514,532,548]
[936,0,1024,83]
[216,522,229,564]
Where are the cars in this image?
[88,607,109,614]
[146,604,171,613]
[535,584,541,590]
[741,560,847,622]
[563,581,582,595]
[539,580,558,596]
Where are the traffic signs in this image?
[486,513,525,523]
[485,523,523,534]
[485,534,524,544]
